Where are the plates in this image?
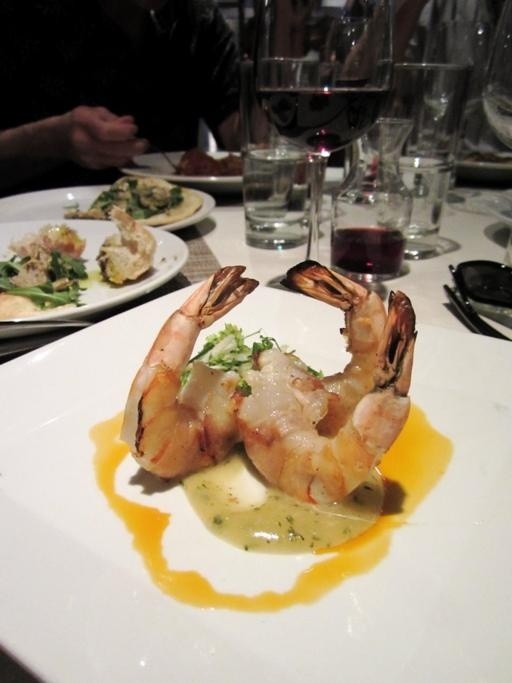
[116,146,242,193]
[1,183,216,231]
[1,217,190,334]
[2,273,512,680]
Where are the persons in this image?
[1,0,271,198]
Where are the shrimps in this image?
[120,265,259,480]
[251,261,387,438]
[241,290,418,504]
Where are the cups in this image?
[240,0,512,295]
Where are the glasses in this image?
[444,259,512,341]
